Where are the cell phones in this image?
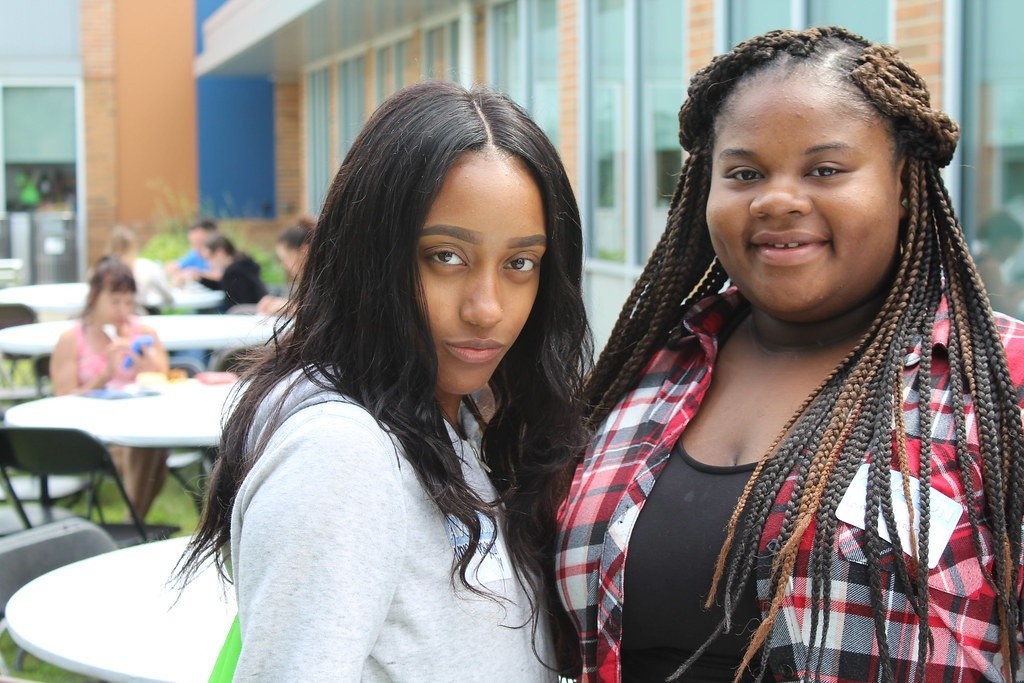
[125,336,154,368]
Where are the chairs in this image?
[0,304,265,682]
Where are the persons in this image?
[167,81,594,681]
[256,217,318,317]
[48,256,171,523]
[162,220,236,291]
[539,25,1024,683]
[72,229,167,317]
[189,237,267,314]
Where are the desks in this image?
[4,533,239,682]
[0,313,295,354]
[4,377,253,446]
[0,283,224,316]
